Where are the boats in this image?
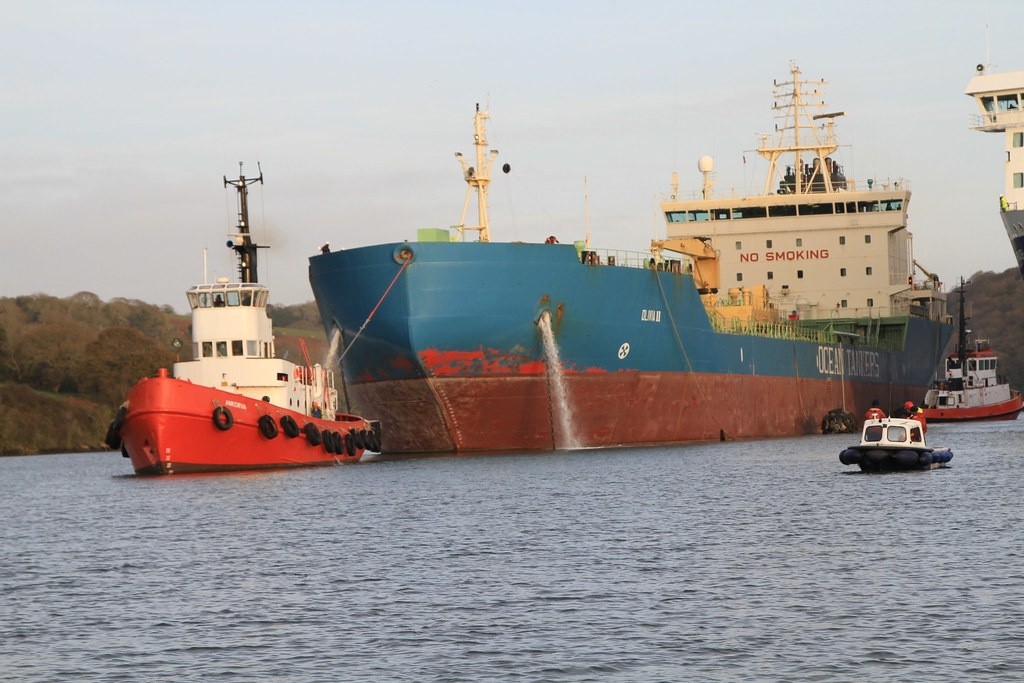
[920,275,1024,423]
[839,417,954,473]
[104,160,383,475]
[308,60,955,454]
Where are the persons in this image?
[865,399,887,434]
[999,193,1009,212]
[898,401,927,441]
[546,236,557,244]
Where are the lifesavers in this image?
[212,406,234,430]
[280,415,301,438]
[104,406,130,458]
[322,429,383,456]
[304,422,322,447]
[259,414,278,439]
